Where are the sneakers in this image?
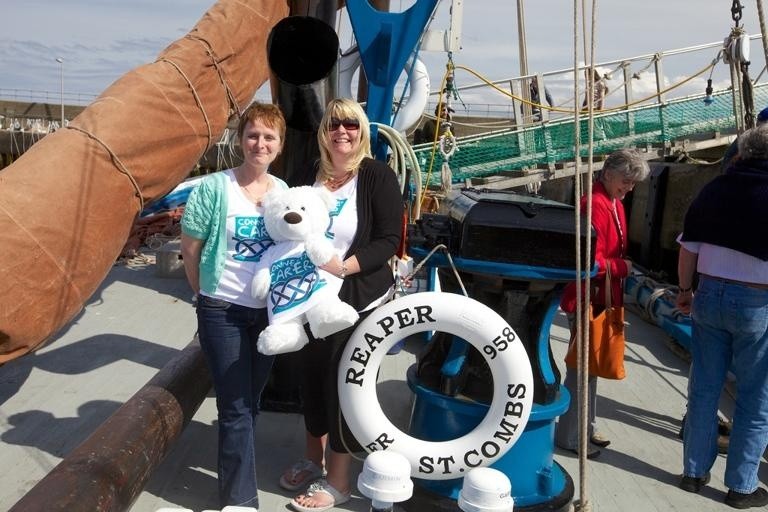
[589,429,610,447]
[555,437,601,459]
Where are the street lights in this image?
[55,57,65,129]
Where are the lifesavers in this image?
[337,291,535,481]
[332,44,429,131]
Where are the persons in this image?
[179,100,288,512]
[673,120,766,509]
[423,102,455,144]
[279,95,405,511]
[554,148,651,459]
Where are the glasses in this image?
[325,117,360,130]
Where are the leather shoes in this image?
[679,409,733,454]
[680,469,711,493]
[724,486,768,508]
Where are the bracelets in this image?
[677,285,691,295]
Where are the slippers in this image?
[290,478,352,511]
[278,454,328,492]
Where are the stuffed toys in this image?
[251,185,359,356]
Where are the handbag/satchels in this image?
[564,260,627,381]
